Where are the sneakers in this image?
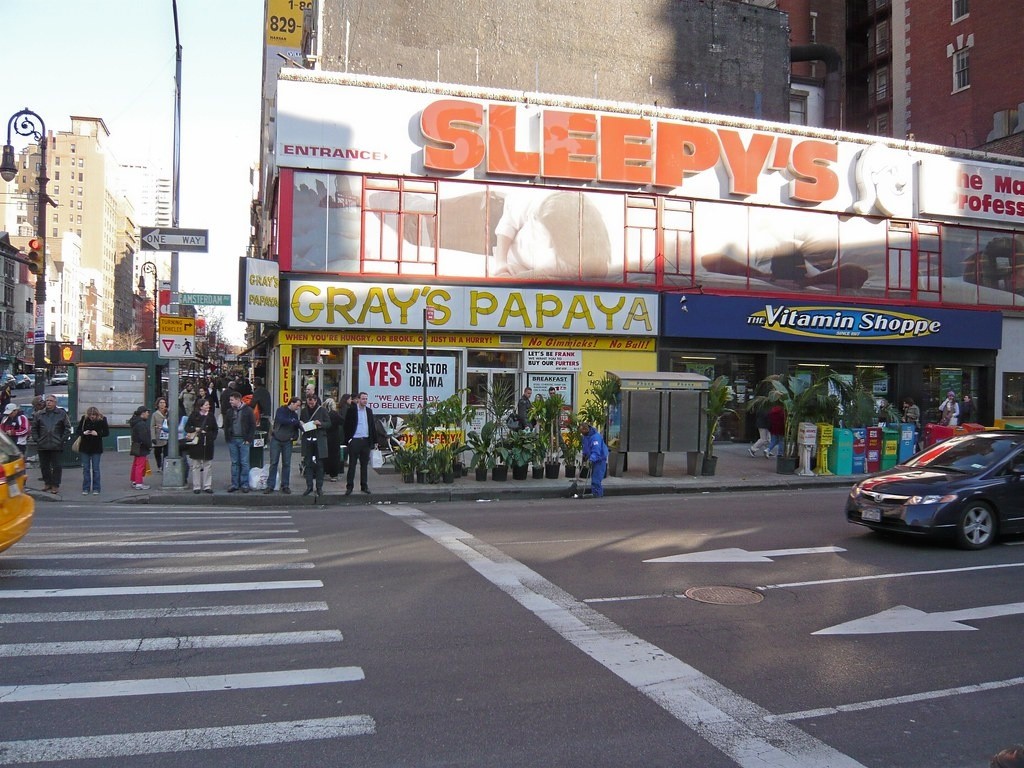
[131,481,150,490]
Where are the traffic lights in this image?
[28,237,44,276]
[61,344,82,363]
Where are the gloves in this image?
[152,439,157,444]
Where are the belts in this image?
[306,437,317,441]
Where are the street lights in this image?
[0,107,47,404]
[137,261,157,348]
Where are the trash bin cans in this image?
[60,420,83,468]
[247,431,267,468]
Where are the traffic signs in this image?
[158,316,197,359]
[138,225,209,253]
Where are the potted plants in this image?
[700,374,742,476]
[392,375,623,484]
[746,368,856,475]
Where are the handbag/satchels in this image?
[507,417,522,431]
[129,442,141,456]
[370,447,383,469]
[71,436,81,454]
[184,436,199,445]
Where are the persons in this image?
[532,394,544,433]
[128,405,152,490]
[264,396,302,494]
[518,387,536,430]
[0,402,31,486]
[545,387,564,449]
[579,422,608,498]
[151,397,168,472]
[184,398,219,494]
[31,394,72,493]
[0,373,16,414]
[223,391,256,493]
[299,382,376,496]
[369,189,612,277]
[938,391,959,426]
[956,395,976,425]
[77,407,110,495]
[901,397,921,452]
[748,404,787,458]
[829,394,888,428]
[162,373,271,482]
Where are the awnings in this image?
[17,357,32,363]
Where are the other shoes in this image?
[93,489,99,494]
[769,451,774,456]
[263,486,274,493]
[41,484,59,494]
[206,489,214,493]
[193,489,201,494]
[243,488,249,492]
[82,489,90,494]
[763,449,769,459]
[228,486,235,492]
[747,448,755,457]
[283,486,291,494]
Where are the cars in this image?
[14,374,32,389]
[51,393,69,413]
[0,427,35,554]
[844,430,1024,550]
[17,403,34,421]
[51,373,69,386]
[0,374,16,391]
[27,374,36,387]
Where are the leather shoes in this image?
[303,487,313,496]
[345,487,353,495]
[361,486,371,493]
[317,487,323,496]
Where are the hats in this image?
[3,403,17,414]
[947,391,955,397]
[137,406,152,412]
